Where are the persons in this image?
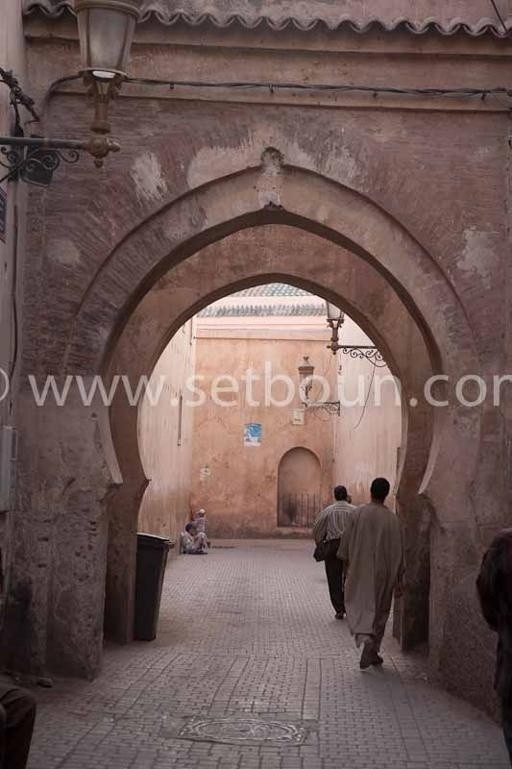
[311,485,360,620]
[0,679,37,769]
[334,476,408,670]
[189,508,207,549]
[473,527,511,763]
[181,523,211,555]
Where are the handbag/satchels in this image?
[313,538,340,562]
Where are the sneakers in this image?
[360,653,383,669]
[334,612,343,620]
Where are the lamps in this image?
[296,356,341,416]
[325,300,386,369]
[0,4,139,184]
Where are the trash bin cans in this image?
[133,532,176,641]
